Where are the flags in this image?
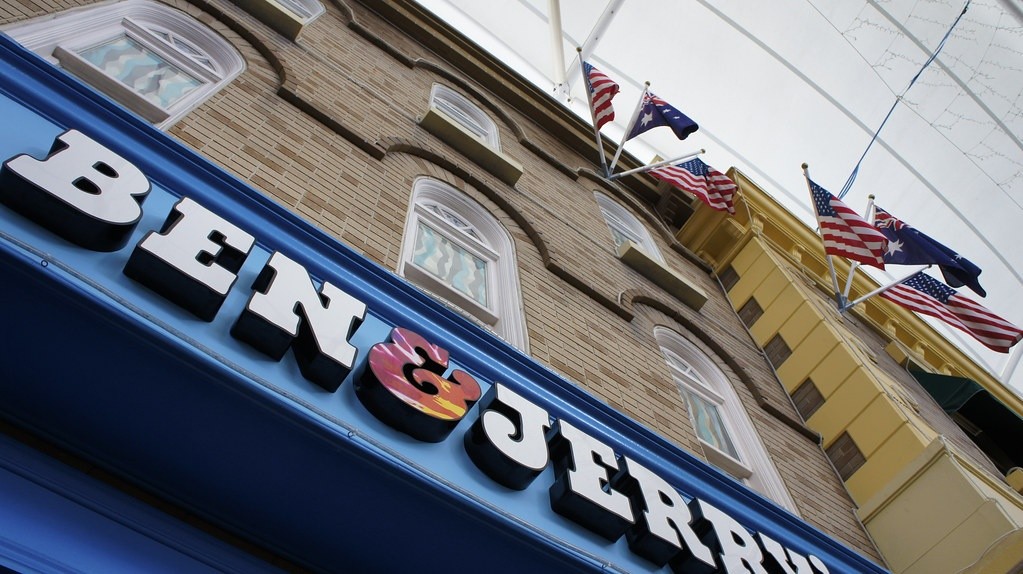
[873,205,987,298]
[649,157,739,210]
[583,60,620,130]
[626,91,699,140]
[808,178,889,271]
[879,272,1023,354]
